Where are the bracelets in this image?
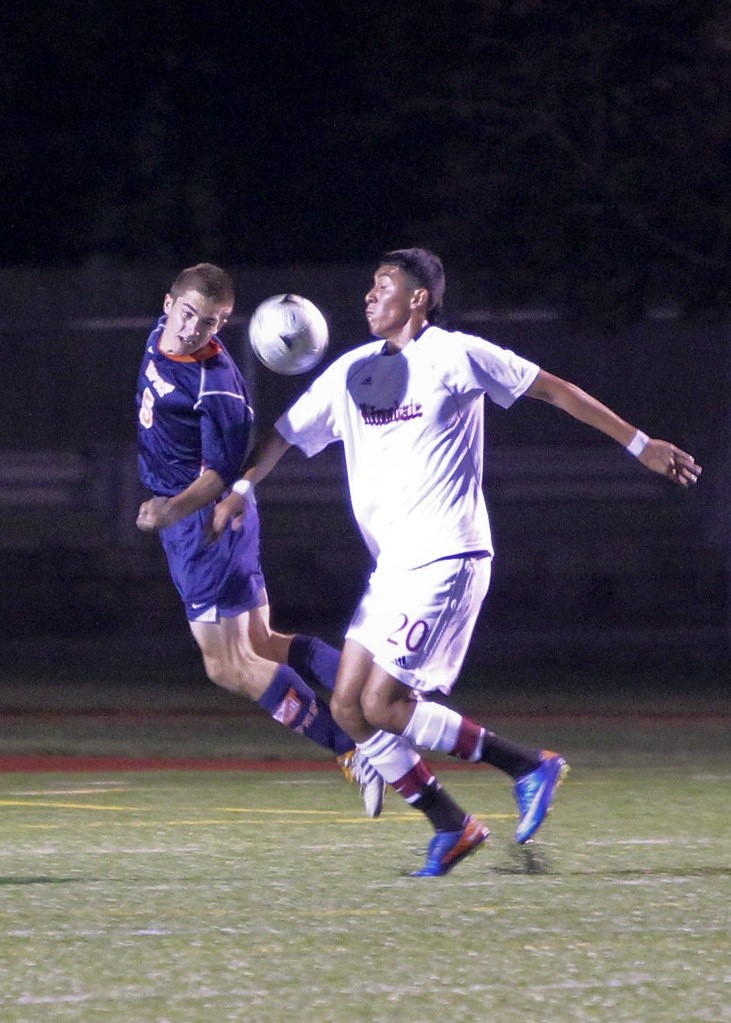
[626,429,649,457]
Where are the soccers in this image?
[248,291,331,377]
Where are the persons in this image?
[135,263,387,819]
[213,248,704,876]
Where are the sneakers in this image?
[337,748,384,818]
[512,749,571,845]
[407,812,491,876]
